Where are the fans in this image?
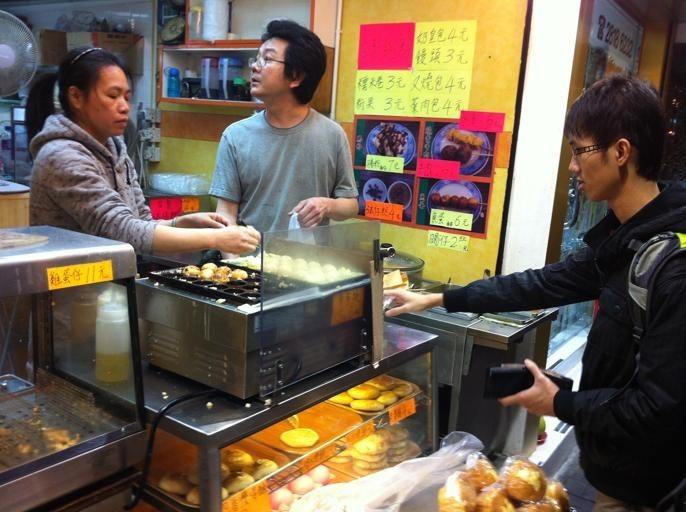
[0,9,40,98]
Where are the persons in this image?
[383,73,684,511]
[205,18,360,260]
[21,46,260,273]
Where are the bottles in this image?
[200,54,242,100]
[164,67,181,98]
[64,280,132,382]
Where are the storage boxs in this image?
[36,27,143,76]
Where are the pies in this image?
[159,374,412,505]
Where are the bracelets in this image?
[172,216,176,227]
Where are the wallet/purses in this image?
[482,362,573,400]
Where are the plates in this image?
[363,178,387,204]
[160,18,185,42]
[367,123,417,167]
[427,179,483,226]
[387,181,413,210]
[430,124,492,176]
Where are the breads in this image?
[438,459,568,512]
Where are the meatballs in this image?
[183,262,247,283]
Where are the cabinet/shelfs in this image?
[152,0,338,115]
[53,319,447,512]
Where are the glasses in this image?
[247,57,286,68]
[568,140,615,160]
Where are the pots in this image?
[375,244,425,290]
